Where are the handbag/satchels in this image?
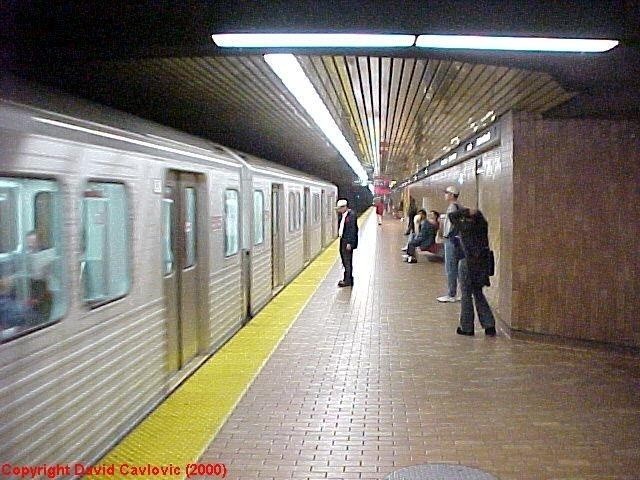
[464,259,490,287]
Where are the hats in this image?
[442,186,460,196]
[334,200,347,209]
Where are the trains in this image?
[0,68,340,480]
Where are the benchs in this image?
[420,243,442,254]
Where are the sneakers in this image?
[437,296,455,302]
[486,327,496,335]
[339,282,351,287]
[457,327,474,336]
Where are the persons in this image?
[376,199,385,225]
[446,206,497,337]
[12,231,53,329]
[332,199,359,285]
[405,198,418,235]
[435,185,466,303]
[401,209,440,264]
